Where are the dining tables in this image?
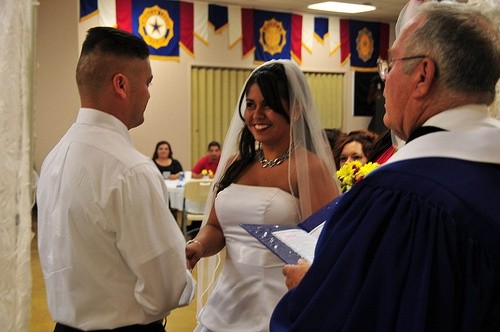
[163,177,214,214]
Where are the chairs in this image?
[181,181,212,243]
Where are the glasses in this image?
[377,54,428,81]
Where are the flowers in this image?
[334,160,380,196]
[202,169,213,176]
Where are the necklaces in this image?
[257,140,300,168]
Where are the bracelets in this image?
[186,239,205,254]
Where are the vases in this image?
[203,176,210,183]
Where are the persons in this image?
[192,141,222,180]
[268,1,500,332]
[187,59,341,332]
[37,27,197,332]
[333,129,379,171]
[152,141,184,222]
